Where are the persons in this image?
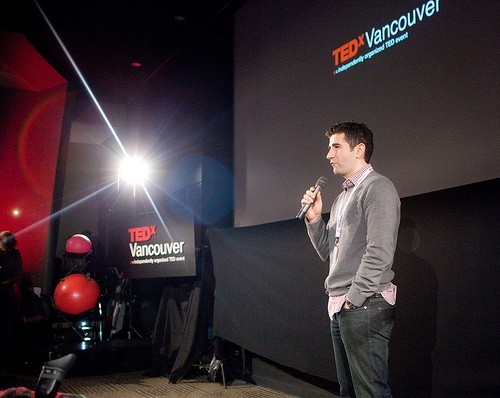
[0,231,23,341]
[300,121,401,398]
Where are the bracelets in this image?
[347,301,356,309]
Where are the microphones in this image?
[295,176,328,221]
